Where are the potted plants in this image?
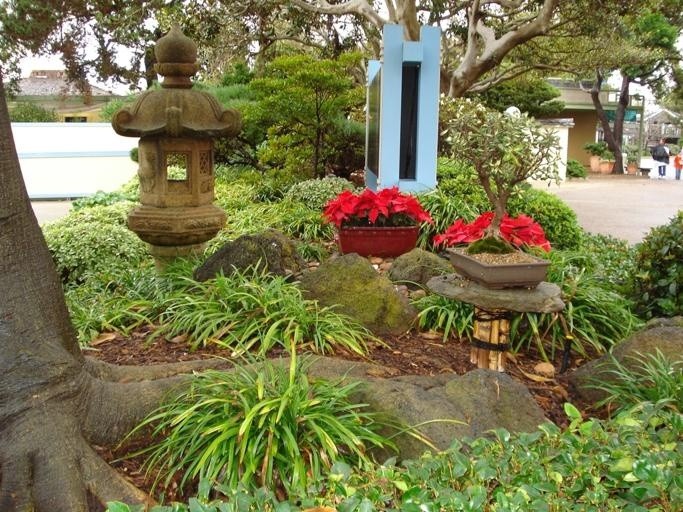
[438,93,570,290]
[583,140,640,176]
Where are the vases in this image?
[334,224,419,258]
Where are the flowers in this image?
[319,185,435,233]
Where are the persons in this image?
[657,138,683,180]
